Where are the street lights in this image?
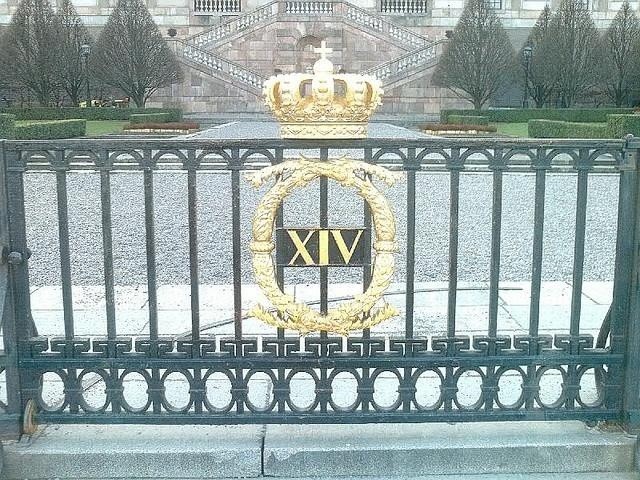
[521,46,533,107]
[80,42,93,107]
